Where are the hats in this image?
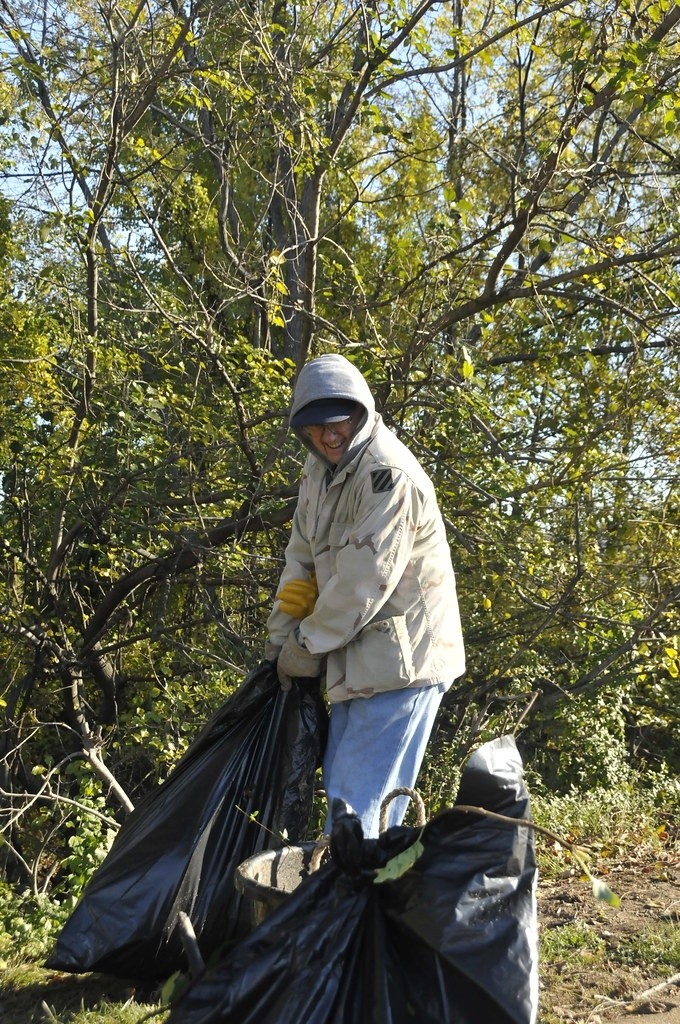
[289,398,357,423]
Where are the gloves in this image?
[264,641,282,664]
[277,626,325,692]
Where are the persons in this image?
[265,349,469,854]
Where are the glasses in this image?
[300,414,355,437]
